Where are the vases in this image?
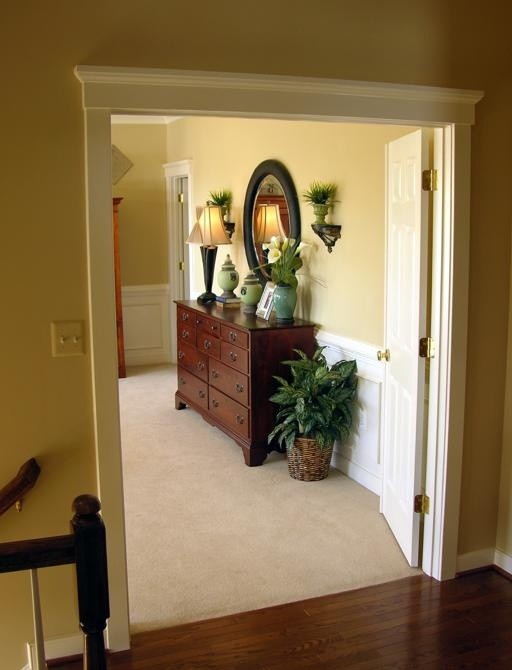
[273,286,298,324]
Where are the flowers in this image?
[261,233,310,286]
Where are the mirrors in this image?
[243,159,302,283]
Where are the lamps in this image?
[184,207,231,304]
[254,202,288,264]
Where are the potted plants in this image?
[210,193,231,221]
[303,182,337,224]
[266,346,361,482]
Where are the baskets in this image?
[285,435,335,482]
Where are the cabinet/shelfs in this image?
[172,300,317,470]
